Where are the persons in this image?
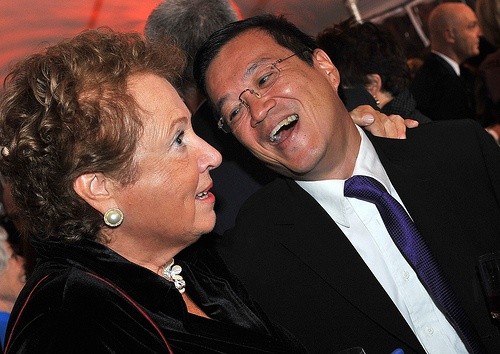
[0,25,419,354]
[316,0,500,148]
[191,14,500,354]
[143,0,237,117]
[0,225,28,353]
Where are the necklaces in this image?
[163,257,186,295]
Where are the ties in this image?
[344,175,485,354]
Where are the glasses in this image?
[218,53,297,134]
